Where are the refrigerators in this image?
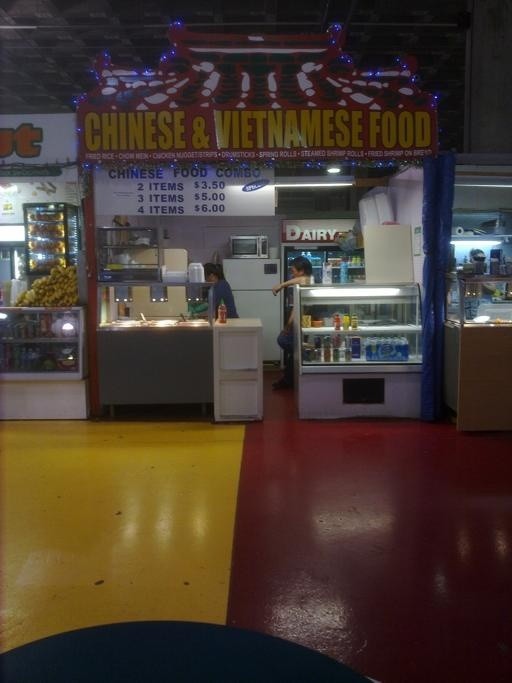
[221,258,283,367]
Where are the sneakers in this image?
[272,375,294,388]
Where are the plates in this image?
[474,315,491,322]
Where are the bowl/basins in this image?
[311,320,323,327]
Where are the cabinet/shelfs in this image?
[452,213,511,320]
[1,308,86,421]
[216,328,265,422]
[303,311,419,365]
[99,227,160,278]
[21,201,79,277]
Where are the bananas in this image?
[15,263,78,308]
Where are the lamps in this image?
[307,288,405,301]
[325,164,346,176]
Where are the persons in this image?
[189,263,238,319]
[271,255,315,389]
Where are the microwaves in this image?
[230,235,269,259]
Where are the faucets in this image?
[136,313,148,323]
[176,312,187,323]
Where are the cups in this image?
[491,256,499,275]
[269,246,278,260]
[302,315,311,328]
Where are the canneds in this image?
[304,335,352,362]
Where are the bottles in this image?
[4,345,42,371]
[334,312,357,330]
[118,302,130,317]
[218,297,229,324]
[287,250,363,284]
[366,335,409,361]
